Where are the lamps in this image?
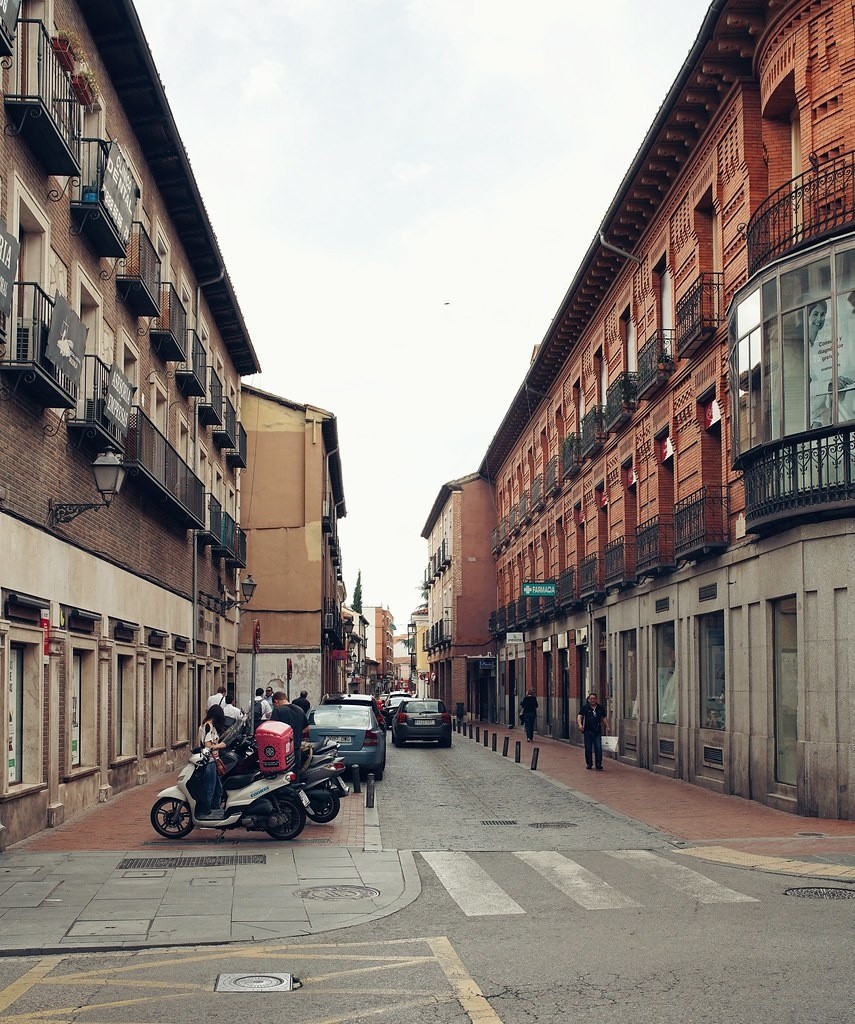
[219,574,257,617]
[48,445,140,530]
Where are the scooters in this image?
[217,707,350,823]
[150,721,315,841]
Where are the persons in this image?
[264,687,274,710]
[520,689,538,742]
[206,687,226,712]
[198,704,226,809]
[223,695,243,720]
[248,688,272,720]
[270,692,310,769]
[577,693,610,771]
[292,691,310,714]
[808,300,854,430]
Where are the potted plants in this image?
[51,27,87,71]
[72,70,99,105]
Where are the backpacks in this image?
[253,699,265,722]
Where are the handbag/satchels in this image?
[600,735,619,753]
[215,759,226,776]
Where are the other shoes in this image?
[586,764,592,769]
[596,765,603,769]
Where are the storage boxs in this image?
[255,721,294,773]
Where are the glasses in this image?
[266,689,271,692]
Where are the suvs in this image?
[319,693,386,738]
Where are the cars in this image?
[388,699,451,748]
[305,705,386,780]
[381,692,412,729]
